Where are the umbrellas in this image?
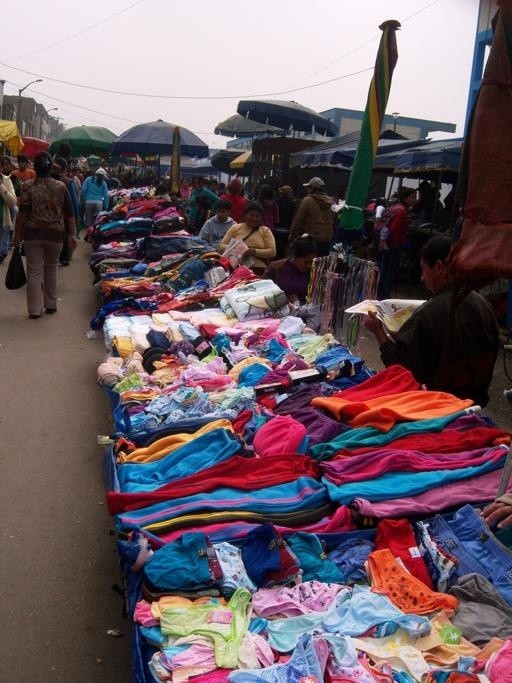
[230,147,280,177]
[237,99,339,185]
[374,141,466,176]
[340,18,399,232]
[17,136,48,163]
[49,125,116,163]
[0,119,23,158]
[215,113,292,199]
[289,128,417,198]
[211,147,252,177]
[114,120,211,192]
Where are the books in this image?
[344,297,429,336]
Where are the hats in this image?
[278,186,293,194]
[303,177,325,189]
[96,168,107,179]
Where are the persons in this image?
[368,197,378,211]
[263,234,318,308]
[219,180,246,221]
[13,150,77,317]
[178,180,189,200]
[50,156,79,266]
[274,187,293,228]
[80,166,109,236]
[12,156,34,188]
[192,196,212,235]
[379,188,418,295]
[1,156,20,241]
[188,177,218,232]
[376,198,387,258]
[200,201,237,251]
[291,178,338,253]
[221,202,277,276]
[0,158,18,264]
[367,236,499,408]
[254,186,278,234]
[67,165,82,203]
[204,178,225,194]
